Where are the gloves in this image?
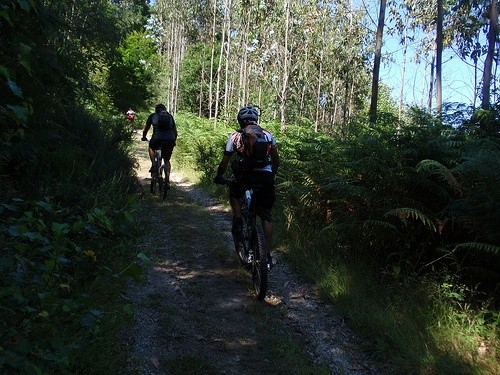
[214,175,224,185]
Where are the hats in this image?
[237,106,258,121]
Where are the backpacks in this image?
[235,125,271,168]
[158,111,172,130]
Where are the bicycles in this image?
[141,138,176,199]
[212,176,274,300]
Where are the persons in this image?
[212,105,280,270]
[126,107,137,133]
[142,103,179,190]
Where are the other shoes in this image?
[231,216,244,234]
[133,131,137,133]
[149,165,159,172]
[265,253,274,269]
[165,182,170,190]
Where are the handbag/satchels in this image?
[233,153,256,174]
[127,114,134,119]
